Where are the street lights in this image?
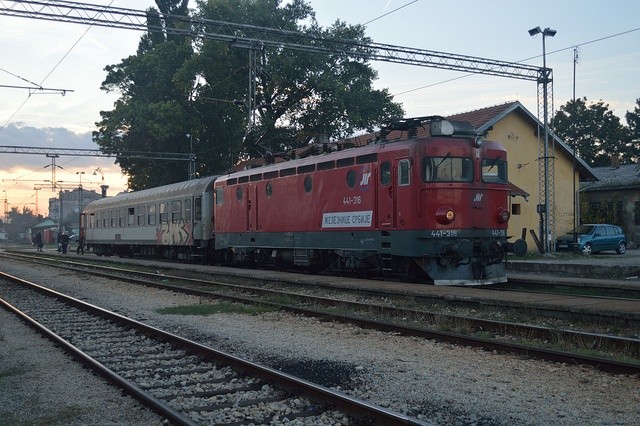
[529,26,557,253]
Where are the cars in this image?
[555,223,627,255]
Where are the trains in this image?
[79,119,527,287]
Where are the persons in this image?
[34,232,44,252]
[76,227,85,255]
[61,231,69,254]
[57,229,62,252]
